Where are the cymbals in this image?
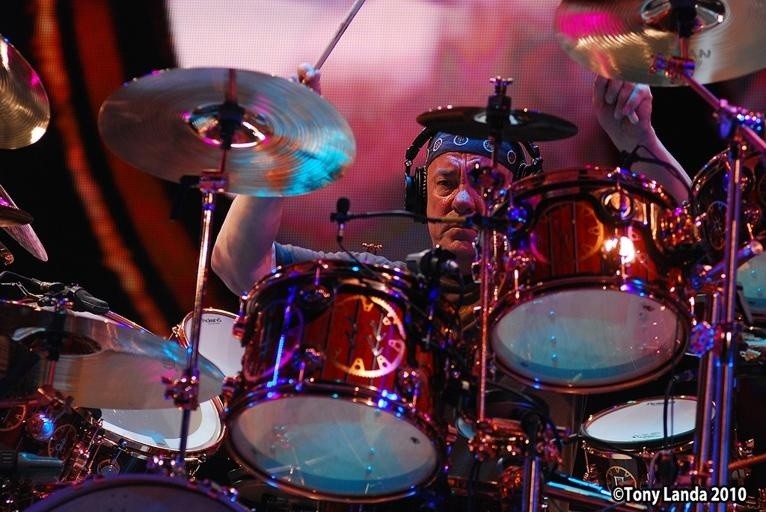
[0,185,50,262]
[554,0,765,88]
[1,34,51,150]
[96,67,357,198]
[1,293,226,409]
[415,105,578,142]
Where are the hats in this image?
[425,131,527,176]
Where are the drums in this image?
[168,307,245,379]
[473,166,695,395]
[579,395,716,508]
[0,395,230,510]
[226,260,455,503]
[20,455,255,512]
[689,145,765,316]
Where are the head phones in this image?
[403,126,546,225]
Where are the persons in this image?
[208,61,695,297]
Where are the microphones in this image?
[335,196,351,243]
